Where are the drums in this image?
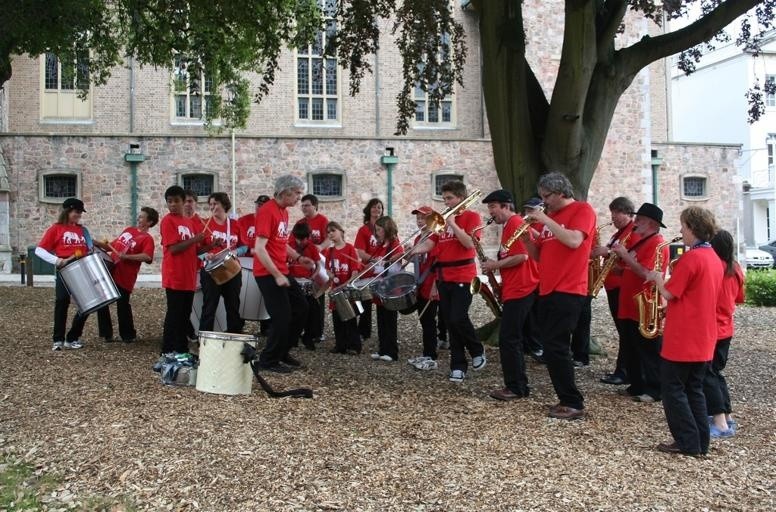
[376,272,417,310]
[329,284,364,321]
[293,277,315,296]
[356,275,381,301]
[205,250,241,285]
[190,271,227,336]
[196,331,258,395]
[237,257,270,319]
[93,240,119,272]
[57,252,122,317]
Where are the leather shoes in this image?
[261,362,291,373]
[282,352,299,366]
[657,441,703,457]
[489,386,521,400]
[550,403,560,411]
[548,406,584,419]
[600,375,623,384]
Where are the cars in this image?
[758,240,776,265]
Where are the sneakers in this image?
[407,357,425,365]
[63,341,83,349]
[415,359,438,371]
[380,355,393,361]
[708,416,735,427]
[631,394,657,402]
[449,369,465,383]
[371,354,379,359]
[472,345,486,371]
[617,388,630,395]
[572,361,583,370]
[709,425,735,437]
[51,341,62,351]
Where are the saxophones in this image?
[589,225,638,298]
[633,236,682,339]
[469,216,505,319]
[588,221,615,299]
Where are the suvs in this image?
[745,247,775,269]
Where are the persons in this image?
[199,193,251,334]
[477,189,540,400]
[588,196,643,385]
[615,202,669,400]
[364,215,403,364]
[353,198,384,342]
[157,184,205,356]
[285,224,316,345]
[34,198,95,352]
[91,207,157,344]
[522,196,547,238]
[236,194,269,259]
[183,188,205,223]
[647,205,724,456]
[403,204,440,372]
[320,221,364,357]
[524,171,597,422]
[429,180,487,382]
[708,230,746,440]
[286,193,329,246]
[253,174,316,374]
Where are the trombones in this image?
[351,189,482,291]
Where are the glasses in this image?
[543,192,554,198]
[63,198,86,212]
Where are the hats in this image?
[628,203,667,229]
[254,195,270,204]
[522,197,544,209]
[411,206,433,215]
[482,190,512,204]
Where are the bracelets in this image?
[295,253,303,264]
